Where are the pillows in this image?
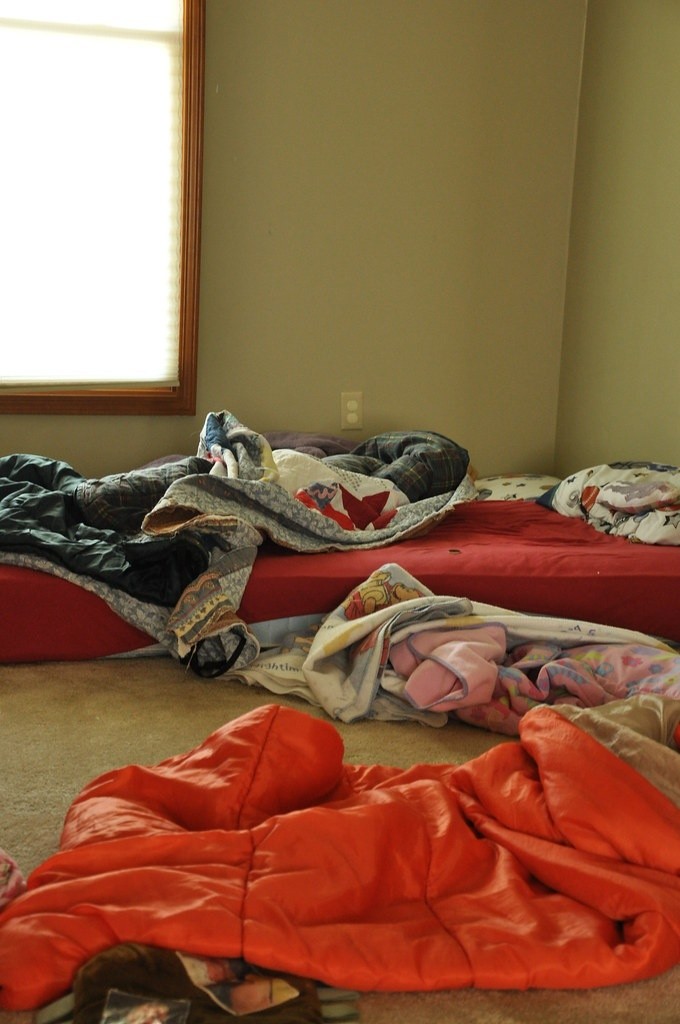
[536,460,680,545]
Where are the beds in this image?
[0,499,680,664]
